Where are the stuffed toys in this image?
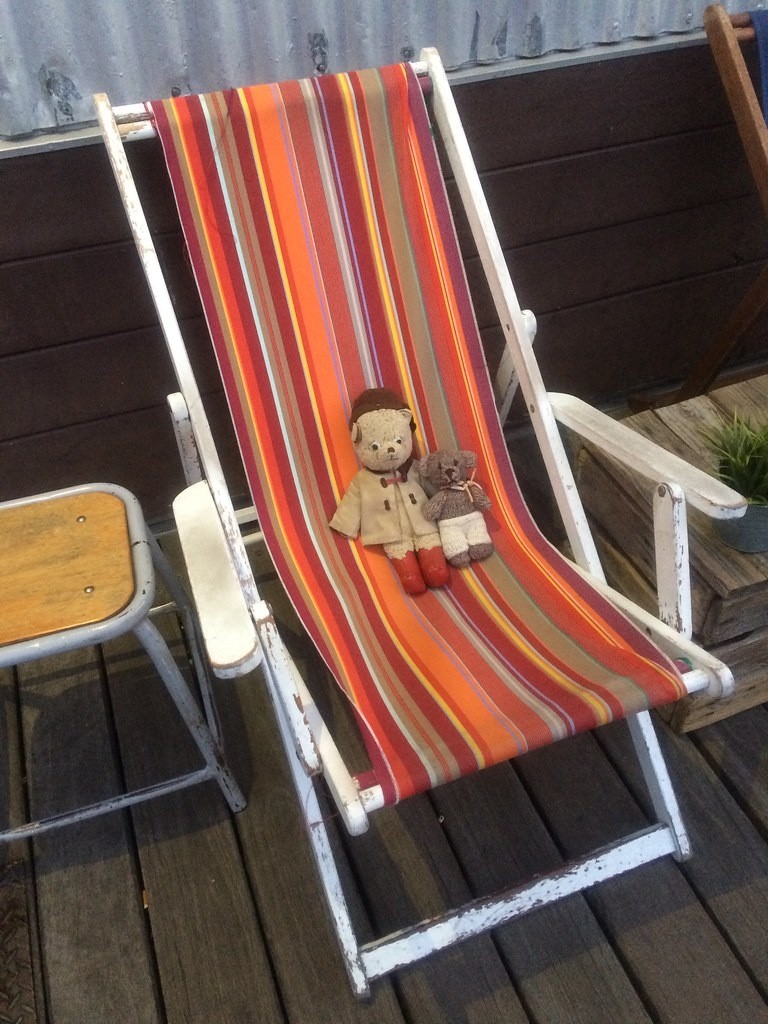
[419,450,493,566]
[328,387,449,594]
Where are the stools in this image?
[0,483,246,843]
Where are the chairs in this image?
[91,48,746,1005]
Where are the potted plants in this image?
[698,404,768,552]
